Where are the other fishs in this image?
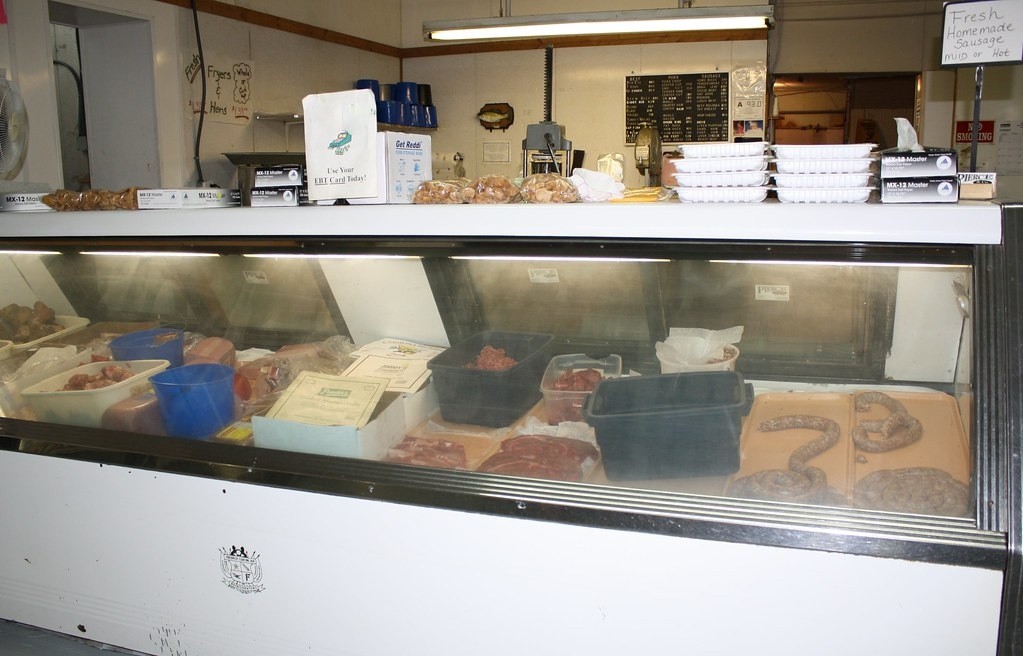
[478,110,513,123]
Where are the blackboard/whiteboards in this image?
[624,72,732,146]
[939,0,1023,66]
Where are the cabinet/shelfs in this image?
[774,81,852,144]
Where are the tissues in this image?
[878,117,960,204]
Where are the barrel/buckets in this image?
[109,328,184,370]
[148,364,233,440]
[356,79,438,128]
[655,343,740,374]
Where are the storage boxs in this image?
[251,377,440,462]
[540,353,623,427]
[427,330,556,427]
[22,359,171,428]
[55,322,161,349]
[582,370,755,481]
[317,131,433,205]
[12,315,90,355]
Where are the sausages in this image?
[726,390,973,518]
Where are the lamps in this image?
[422,4,776,40]
